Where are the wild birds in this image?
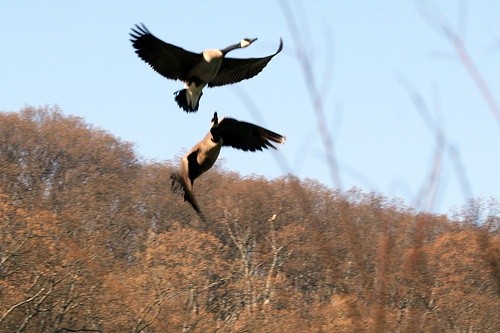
[128,22,283,113]
[169,112,286,221]
